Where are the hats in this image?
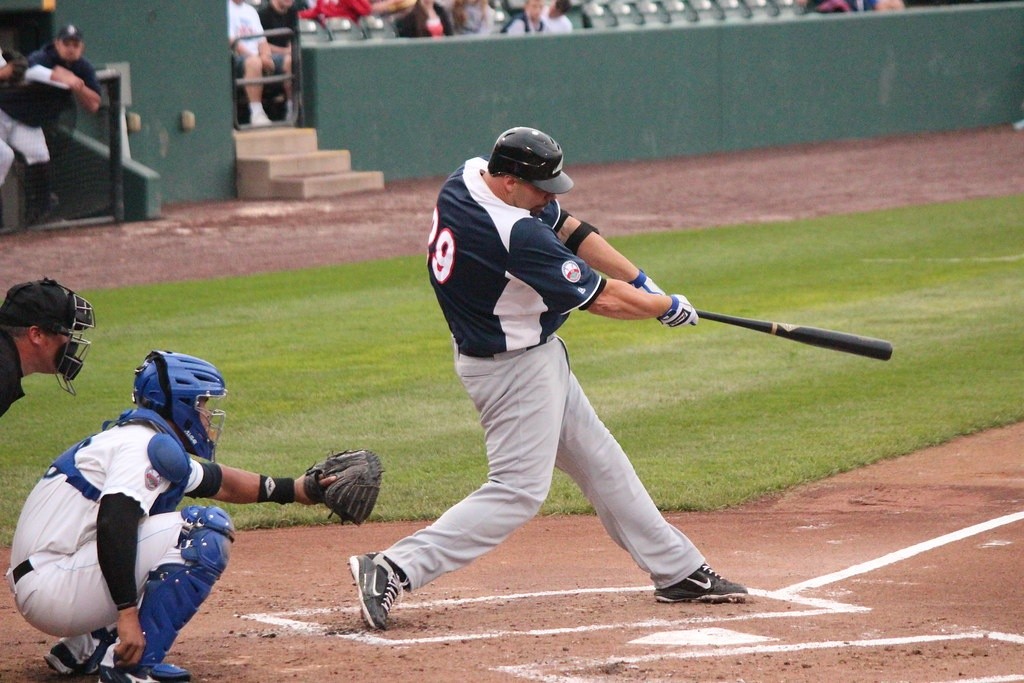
[60,24,85,42]
[0,279,75,331]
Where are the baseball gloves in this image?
[303,449,385,524]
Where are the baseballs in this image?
[695,309,893,362]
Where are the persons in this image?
[228,0,572,127]
[800,0,906,13]
[349,125,747,629]
[0,275,96,421]
[1,25,102,226]
[7,349,383,683]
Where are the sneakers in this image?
[43,642,104,675]
[654,561,748,602]
[98,660,159,683]
[349,554,407,630]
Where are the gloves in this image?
[657,294,699,326]
[627,269,666,297]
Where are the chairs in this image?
[294,15,398,46]
[488,1,805,35]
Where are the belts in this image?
[458,336,546,358]
[12,559,33,584]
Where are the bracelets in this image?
[258,474,294,504]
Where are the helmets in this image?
[131,350,225,461]
[488,127,574,194]
